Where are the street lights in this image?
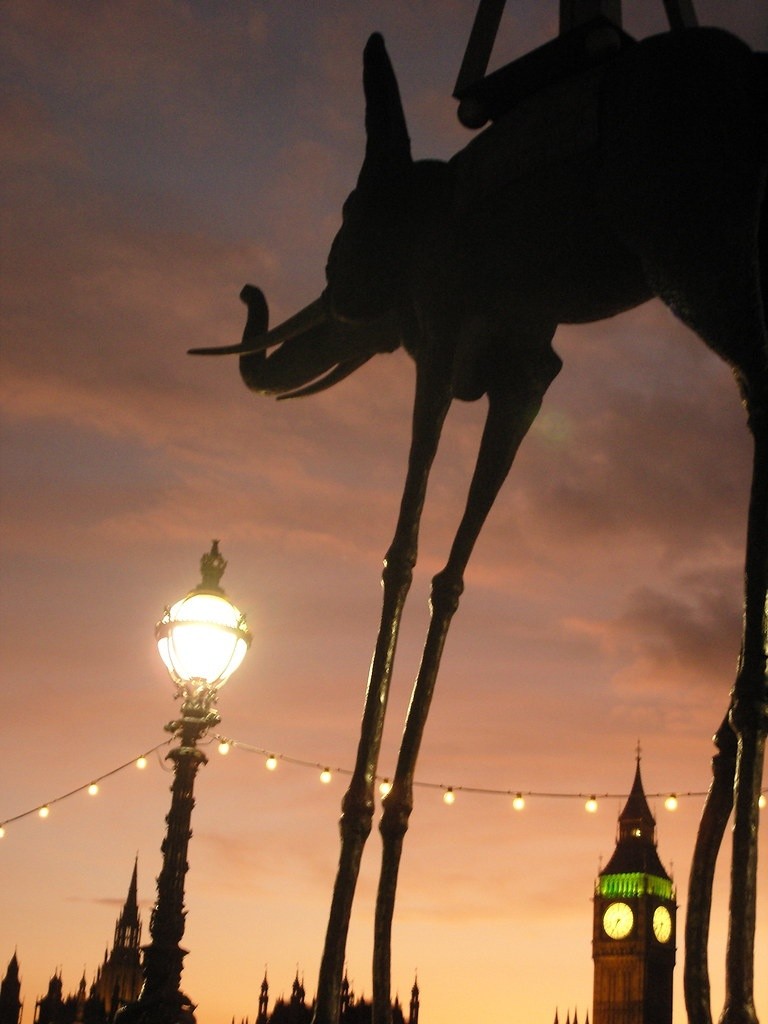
[101,533,251,1024]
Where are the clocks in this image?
[601,902,637,942]
[652,905,673,945]
